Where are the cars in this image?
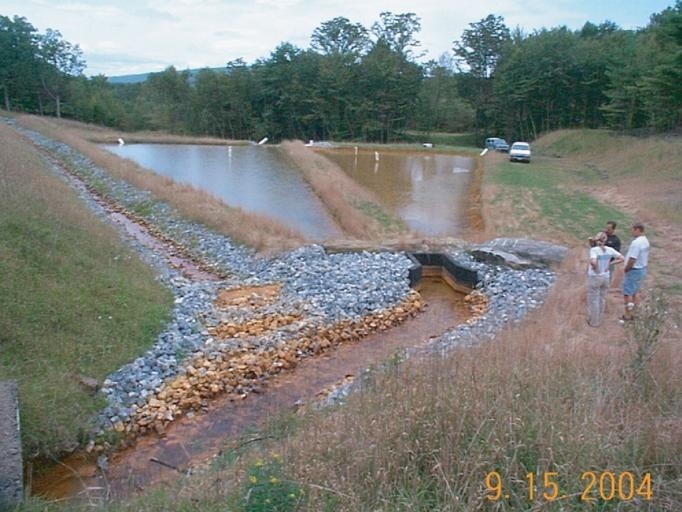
[485,137,531,163]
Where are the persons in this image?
[584,231,625,328]
[615,221,651,325]
[586,220,621,287]
